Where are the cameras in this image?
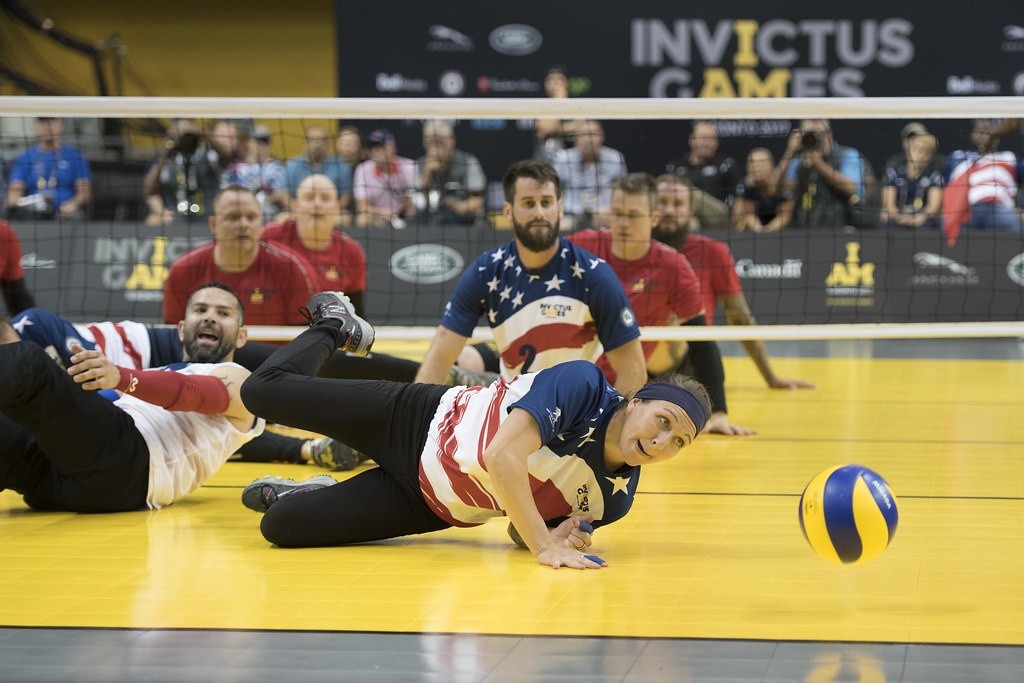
[801,131,822,149]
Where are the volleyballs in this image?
[797,464,899,566]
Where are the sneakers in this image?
[299,291,376,356]
[448,366,498,387]
[241,475,338,513]
[311,437,360,471]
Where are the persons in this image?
[241,290,712,569]
[0,74,1024,475]
[0,282,265,514]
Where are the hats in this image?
[368,130,394,144]
